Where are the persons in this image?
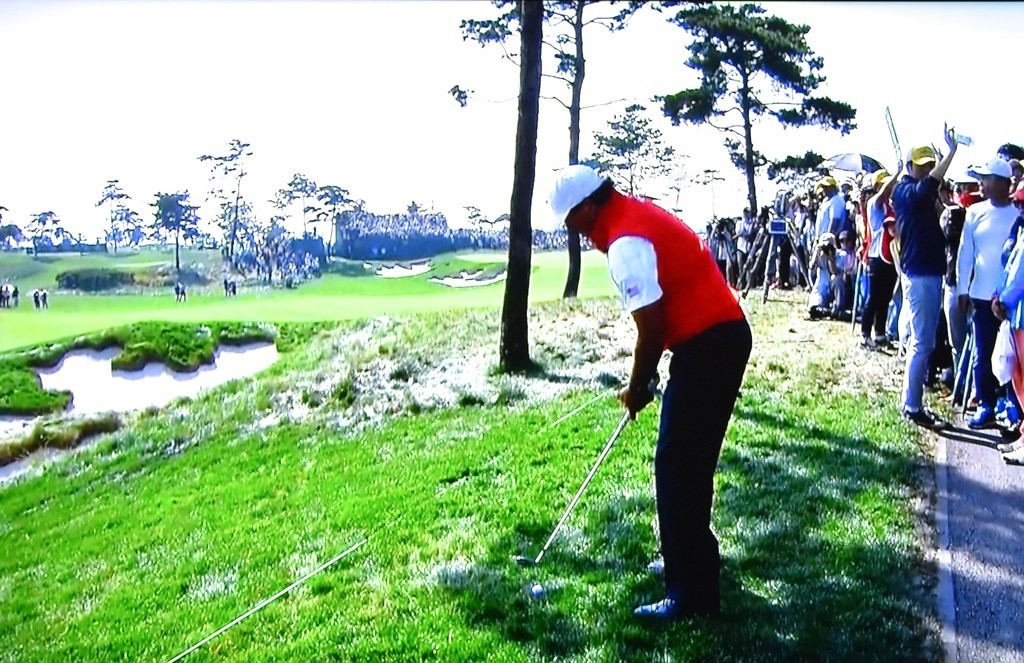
[175,282,186,302]
[0,286,19,308]
[548,164,753,618]
[34,289,48,310]
[224,279,236,297]
[702,119,1024,466]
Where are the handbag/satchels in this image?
[991,318,1017,387]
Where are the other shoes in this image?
[861,338,881,351]
[903,405,946,428]
[875,334,898,354]
[1004,444,1024,465]
[997,435,1024,454]
[968,405,996,429]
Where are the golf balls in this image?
[532,584,543,596]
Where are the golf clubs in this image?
[511,371,661,568]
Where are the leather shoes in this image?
[647,555,665,579]
[634,594,723,621]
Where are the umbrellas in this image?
[817,152,886,177]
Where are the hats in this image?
[817,233,836,247]
[871,169,894,187]
[973,158,1013,178]
[551,165,604,231]
[816,176,837,190]
[906,145,938,165]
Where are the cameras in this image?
[821,244,835,257]
